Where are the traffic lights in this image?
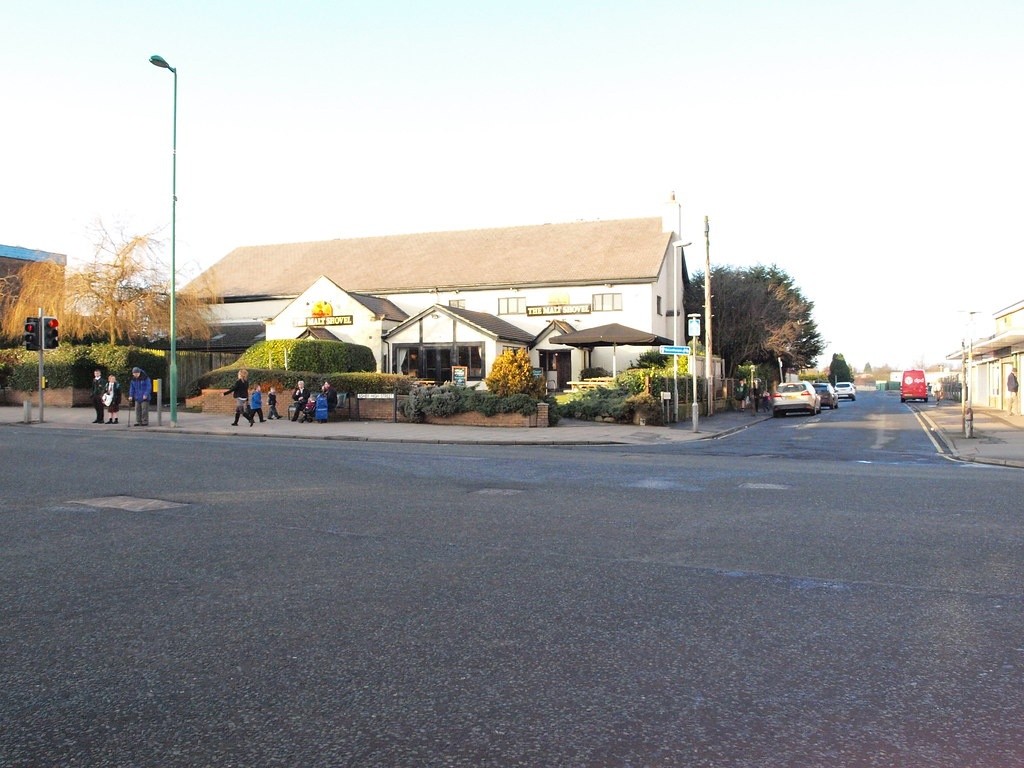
[24,317,39,351]
[42,316,59,352]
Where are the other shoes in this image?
[250,422,255,427]
[291,419,296,421]
[134,424,139,426]
[231,423,238,426]
[99,420,104,424]
[268,417,273,419]
[277,416,281,419]
[260,420,266,422]
[141,424,147,426]
[249,420,255,423]
[92,419,100,423]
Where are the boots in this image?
[112,418,119,424]
[105,418,113,424]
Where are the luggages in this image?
[316,393,328,422]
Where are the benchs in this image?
[563,390,578,393]
[288,393,351,422]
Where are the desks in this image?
[567,377,613,393]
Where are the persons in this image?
[267,387,283,419]
[90,370,107,423]
[291,380,310,422]
[128,367,152,426]
[309,381,337,417]
[933,379,942,405]
[98,375,122,424]
[734,379,771,413]
[223,369,254,427]
[1007,368,1022,416]
[250,383,267,423]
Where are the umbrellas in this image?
[548,323,673,377]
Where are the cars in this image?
[772,380,821,417]
[835,382,856,401]
[813,383,838,410]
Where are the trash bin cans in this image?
[876,380,900,391]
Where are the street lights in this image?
[672,240,691,423]
[149,55,177,429]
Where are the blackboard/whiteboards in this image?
[452,366,468,387]
[532,367,543,377]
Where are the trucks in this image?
[899,369,928,403]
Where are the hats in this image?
[132,367,140,373]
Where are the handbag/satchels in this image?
[101,395,112,406]
[245,402,254,417]
